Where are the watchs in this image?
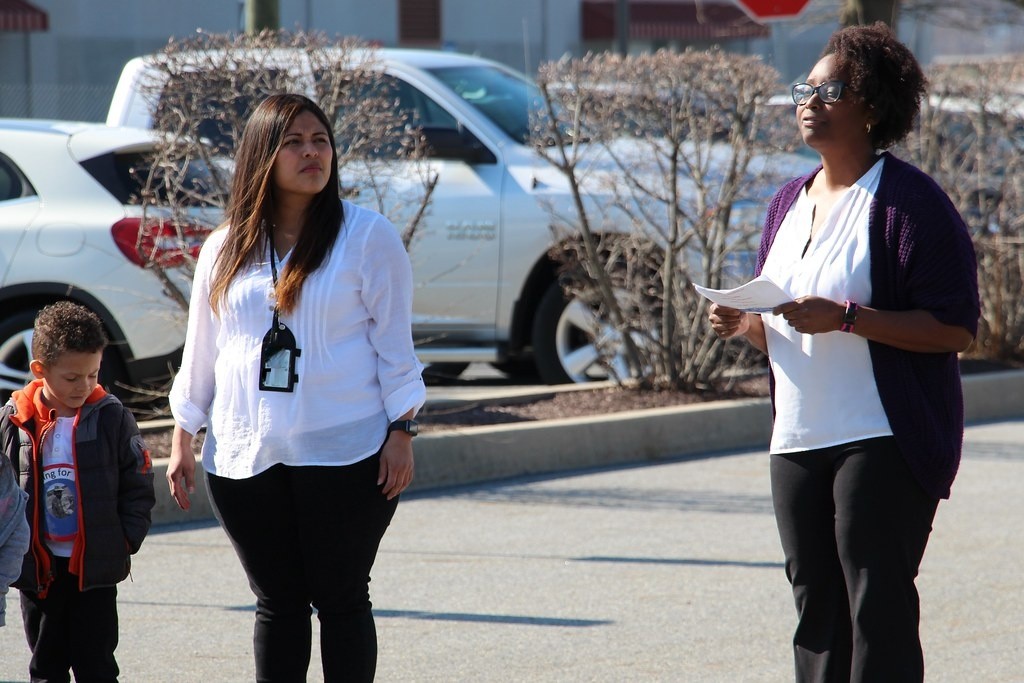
[388,419,420,436]
[841,298,857,334]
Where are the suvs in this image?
[0,115,237,423]
[106,42,783,390]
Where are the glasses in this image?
[792,81,850,105]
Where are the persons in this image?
[1,451,32,627]
[165,93,428,682]
[689,19,981,682]
[0,301,157,683]
